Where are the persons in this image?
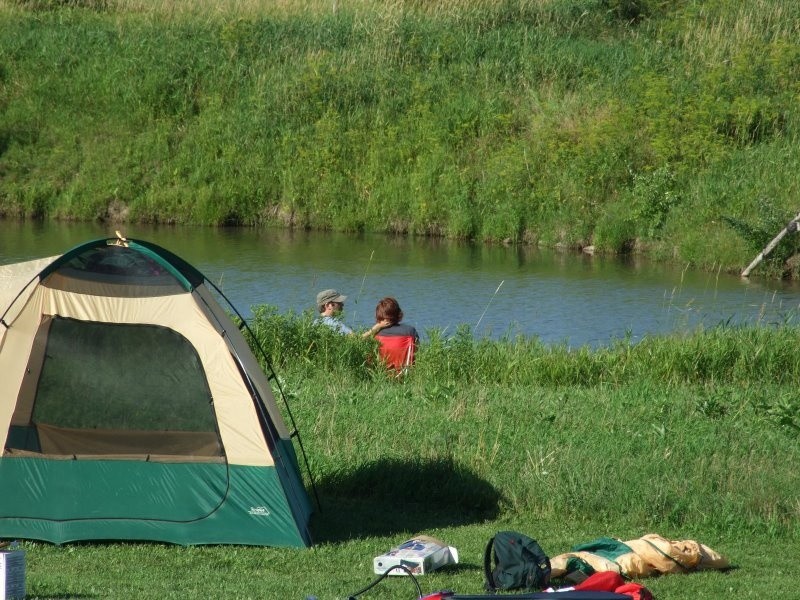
[367,297,421,379]
[313,289,392,341]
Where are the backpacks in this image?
[482,530,552,593]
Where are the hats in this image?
[317,289,347,306]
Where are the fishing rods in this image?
[351,250,375,331]
[473,279,505,333]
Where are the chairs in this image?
[372,335,416,378]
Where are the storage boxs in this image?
[0,551,25,600]
[373,534,460,576]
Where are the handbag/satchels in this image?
[373,535,459,577]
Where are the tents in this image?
[1,231,323,550]
